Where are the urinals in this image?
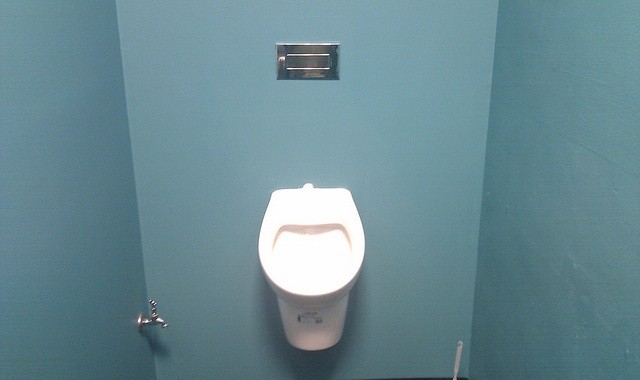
[257,187,366,351]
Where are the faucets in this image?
[138,299,168,330]
